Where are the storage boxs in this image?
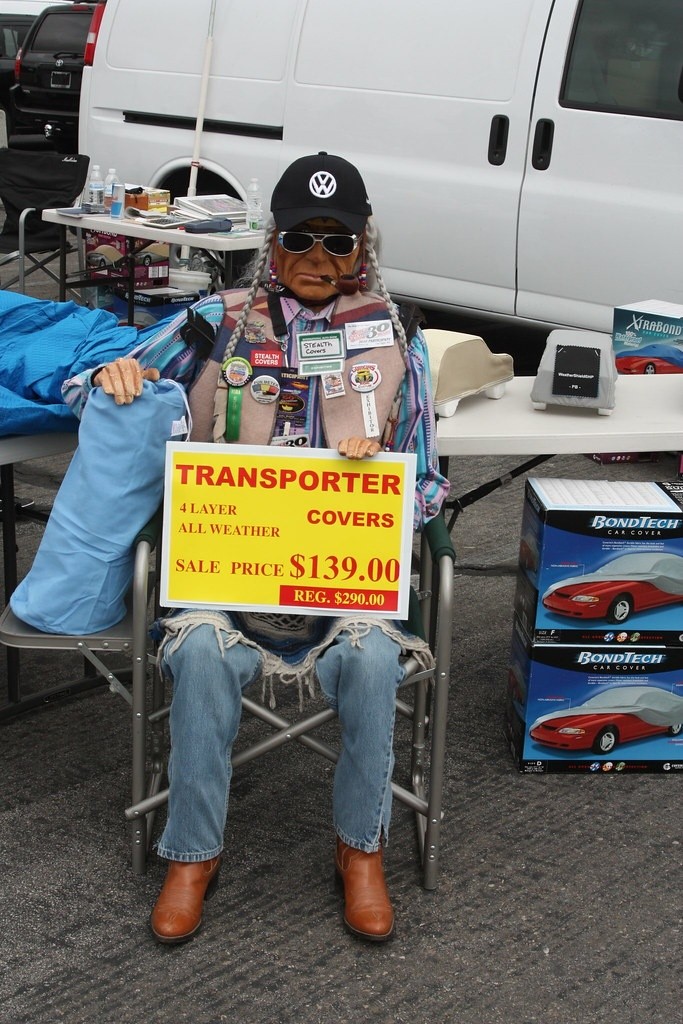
[103,183,170,213]
[583,452,656,466]
[81,228,221,326]
[612,299,683,374]
[502,477,683,774]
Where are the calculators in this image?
[143,218,196,229]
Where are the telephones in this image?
[185,219,234,234]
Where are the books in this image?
[169,194,264,223]
[57,207,112,218]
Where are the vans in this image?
[65,0,683,349]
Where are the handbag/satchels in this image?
[9,378,191,635]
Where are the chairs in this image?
[0,148,90,302]
[126,506,458,888]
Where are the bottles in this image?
[89,165,105,213]
[247,179,265,232]
[104,169,120,211]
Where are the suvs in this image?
[0,11,38,105]
[9,1,104,139]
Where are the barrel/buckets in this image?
[169,269,212,311]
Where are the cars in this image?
[530,686,683,756]
[541,551,682,625]
[615,342,683,375]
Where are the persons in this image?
[62,149,448,943]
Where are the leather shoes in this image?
[335,834,395,941]
[151,853,221,946]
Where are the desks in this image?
[423,374,683,739]
[42,207,266,327]
[0,431,149,721]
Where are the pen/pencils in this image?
[177,227,184,230]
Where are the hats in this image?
[270,151,372,233]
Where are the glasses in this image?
[276,228,364,257]
